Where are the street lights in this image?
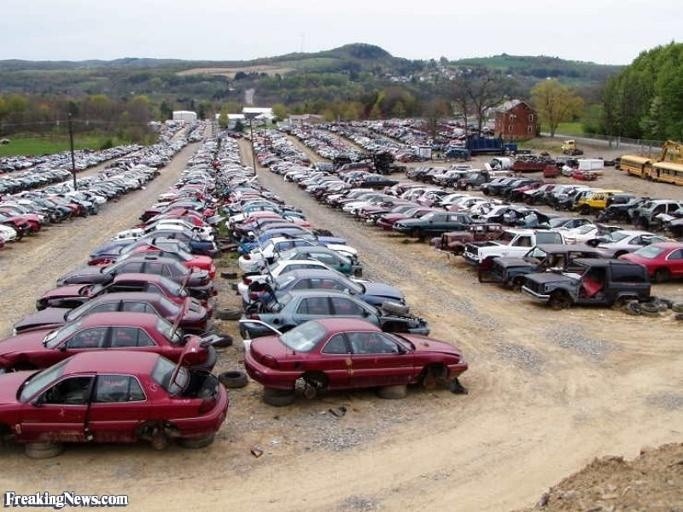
[243,112,260,177]
[64,101,80,191]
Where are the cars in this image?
[0,349,229,450]
[239,289,430,340]
[0,311,216,373]
[242,318,467,402]
[13,291,213,341]
